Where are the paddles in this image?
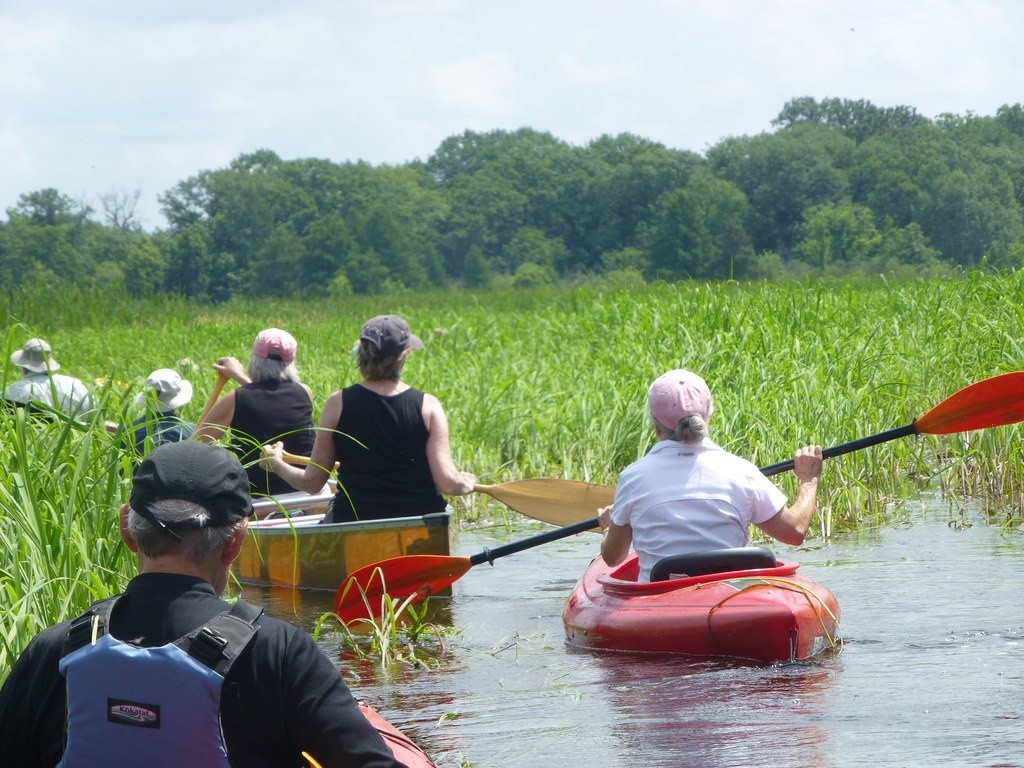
[193,373,228,431]
[266,442,617,535]
[334,371,1024,627]
[1,397,113,438]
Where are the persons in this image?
[122,368,198,453]
[598,370,822,582]
[0,439,395,768]
[259,314,475,523]
[6,337,104,421]
[192,327,317,444]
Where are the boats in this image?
[231,477,456,602]
[560,550,842,662]
[304,701,437,768]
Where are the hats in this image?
[10,338,60,372]
[648,369,713,430]
[128,441,253,542]
[134,369,193,412]
[359,314,423,353]
[252,328,297,361]
